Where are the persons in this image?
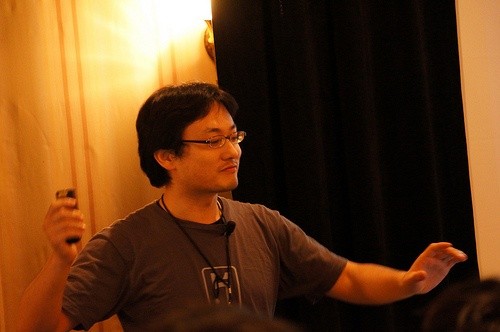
[196,309,298,332]
[16,79,468,332]
[421,277,500,332]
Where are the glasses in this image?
[170,131,245,148]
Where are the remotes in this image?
[56,188,82,244]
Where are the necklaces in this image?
[160,191,239,305]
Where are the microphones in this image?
[224,220,235,237]
